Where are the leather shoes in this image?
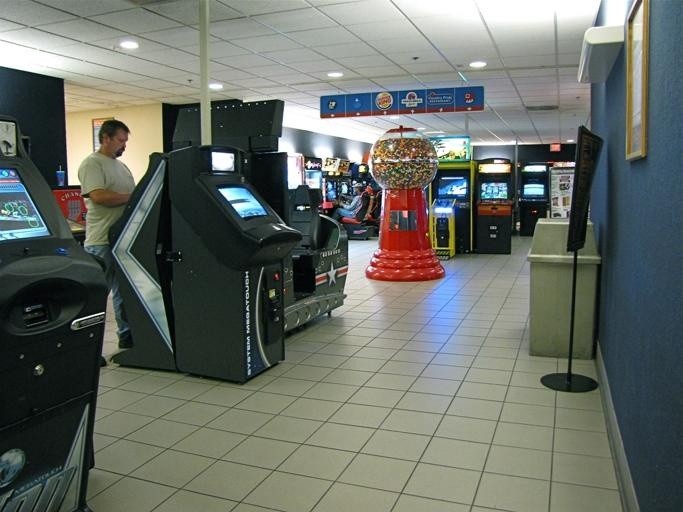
[119,330,133,348]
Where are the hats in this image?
[365,186,373,195]
[352,183,363,188]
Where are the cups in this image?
[56,170,66,186]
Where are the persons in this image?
[78,120,135,366]
[330,182,363,221]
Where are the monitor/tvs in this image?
[327,182,349,201]
[523,183,544,196]
[437,177,470,200]
[218,186,268,221]
[0,169,52,243]
[480,182,508,200]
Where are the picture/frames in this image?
[624,2,649,164]
[91,117,114,153]
